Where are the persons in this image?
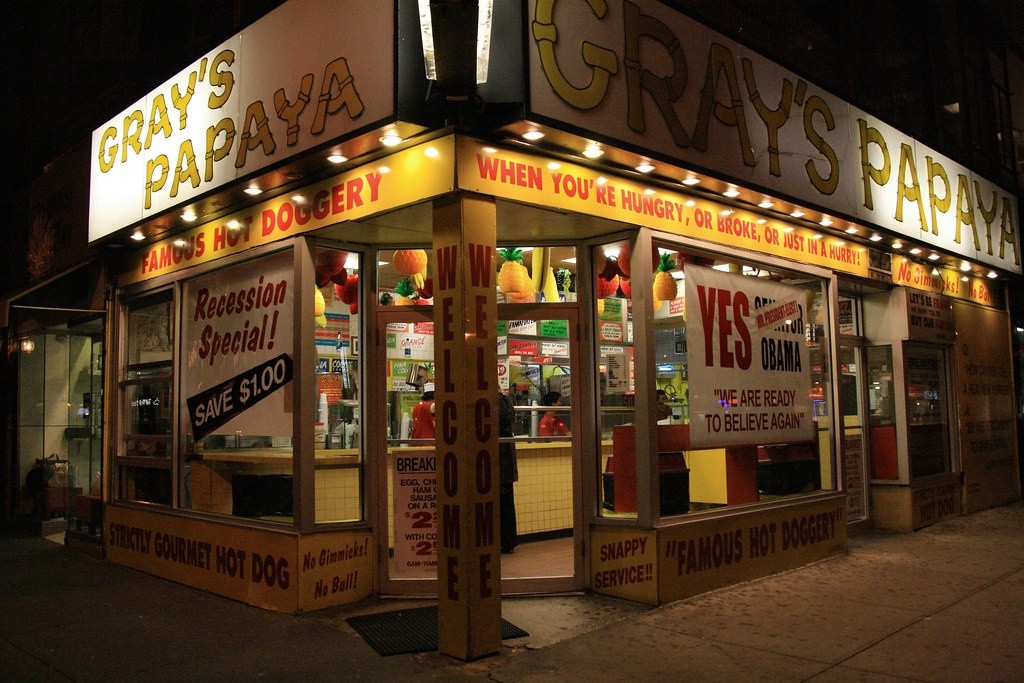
[657,388,672,418]
[537,392,571,443]
[409,384,436,447]
[497,384,519,554]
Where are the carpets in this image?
[344,602,530,657]
